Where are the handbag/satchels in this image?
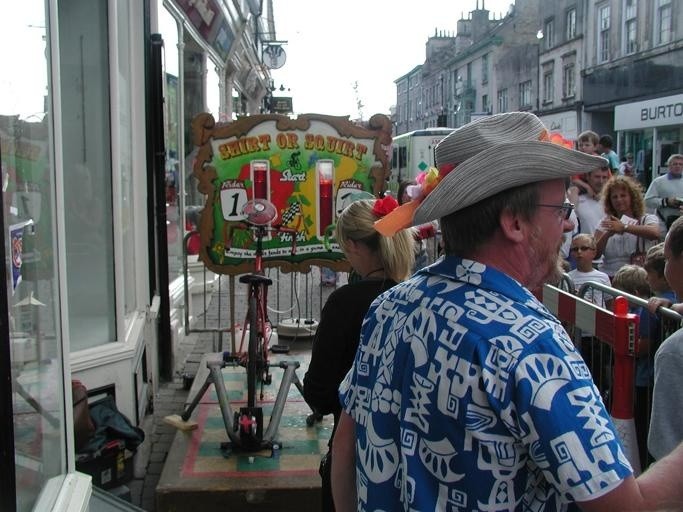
[631,251,647,267]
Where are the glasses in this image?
[574,246,594,252]
[528,200,576,219]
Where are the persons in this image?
[398,180,439,275]
[554,131,682,461]
[303,195,413,510]
[330,112,683,510]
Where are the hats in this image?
[412,110,608,225]
